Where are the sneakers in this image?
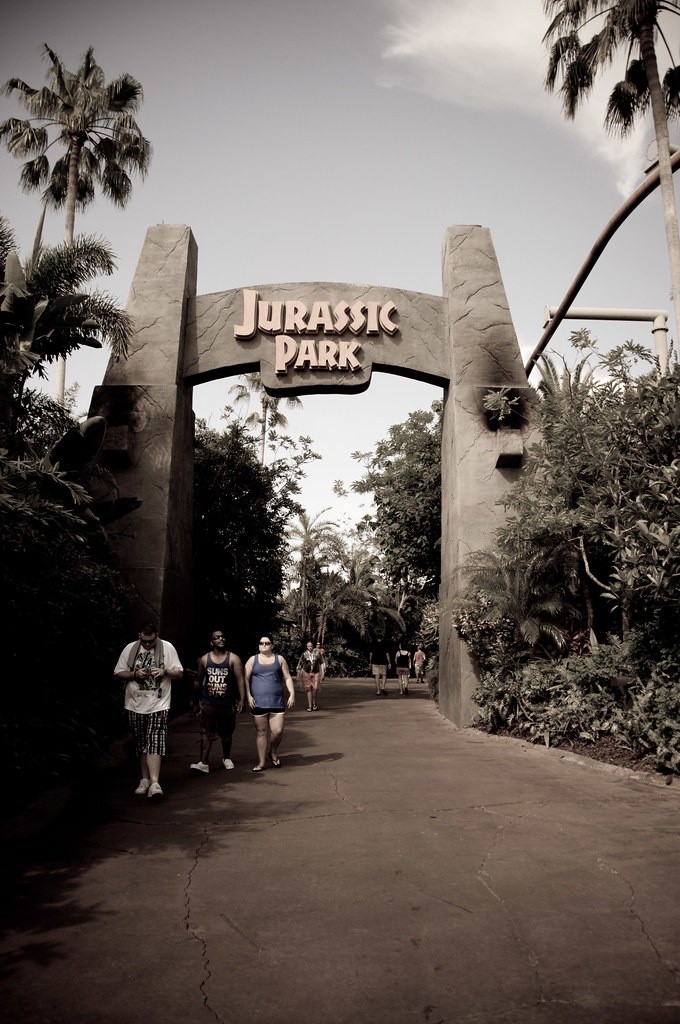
[135,778,151,794]
[148,782,163,797]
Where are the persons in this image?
[369,634,391,695]
[395,642,412,696]
[242,635,295,772]
[113,625,183,798]
[191,630,244,773]
[297,640,326,711]
[413,644,426,683]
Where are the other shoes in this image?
[313,704,317,710]
[405,687,408,695]
[398,693,403,695]
[190,762,209,773]
[222,758,234,769]
[382,689,388,695]
[376,694,380,696]
[307,706,312,712]
[417,680,419,683]
[421,681,424,683]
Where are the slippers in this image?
[252,765,268,772]
[268,751,281,768]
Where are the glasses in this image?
[212,635,224,641]
[259,642,271,645]
[140,636,156,643]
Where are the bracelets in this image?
[134,670,136,677]
